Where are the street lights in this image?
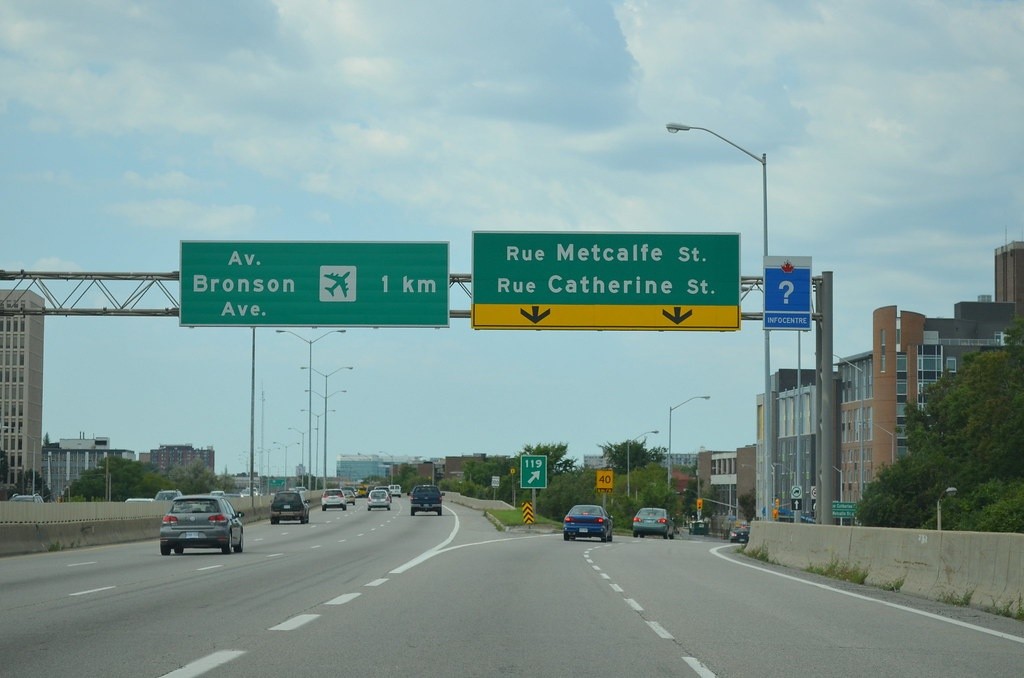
[774,463,797,524]
[627,430,659,498]
[1,426,36,493]
[858,421,894,463]
[937,488,959,530]
[668,396,709,490]
[741,464,764,521]
[239,329,354,491]
[665,123,774,532]
[712,467,731,515]
[380,451,393,485]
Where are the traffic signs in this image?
[472,231,742,331]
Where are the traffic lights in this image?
[696,498,702,510]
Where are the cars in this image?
[341,488,355,505]
[563,505,613,542]
[367,487,391,510]
[728,525,750,542]
[354,485,374,497]
[212,487,260,497]
[633,507,675,539]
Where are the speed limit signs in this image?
[596,471,614,493]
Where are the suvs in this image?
[270,491,310,523]
[154,490,182,501]
[160,495,244,553]
[408,486,445,516]
[321,490,347,511]
[10,494,44,503]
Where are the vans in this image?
[389,485,402,497]
